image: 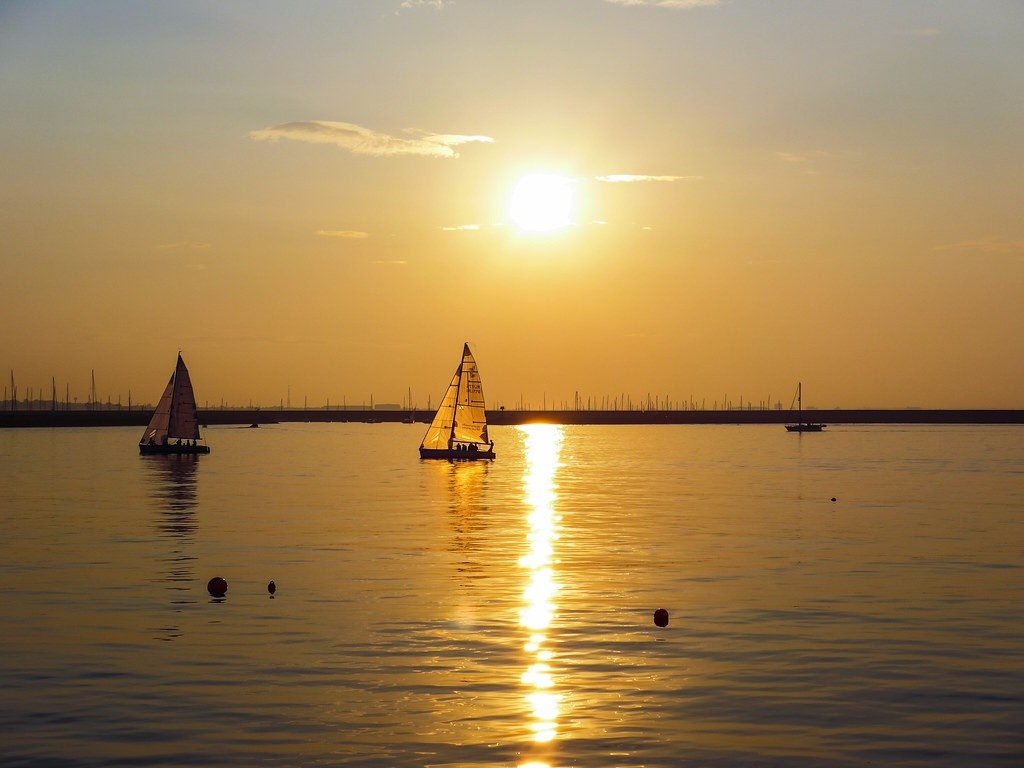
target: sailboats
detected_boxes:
[137,351,212,454]
[418,342,497,461]
[783,381,827,432]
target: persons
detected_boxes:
[488,440,493,452]
[176,438,197,446]
[148,438,158,446]
[456,442,478,452]
